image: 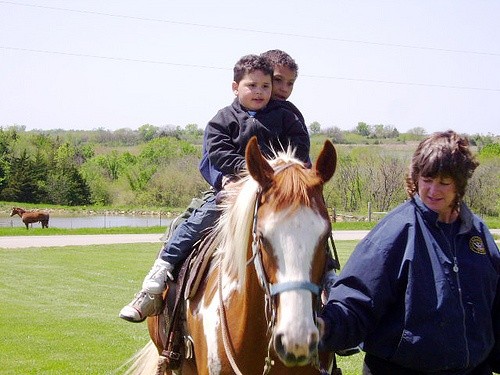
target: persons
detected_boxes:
[160,50,361,356]
[119,55,310,324]
[339,129,500,375]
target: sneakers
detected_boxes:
[141,258,175,294]
[119,293,162,322]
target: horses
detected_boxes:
[10,207,50,229]
[146,136,339,374]
